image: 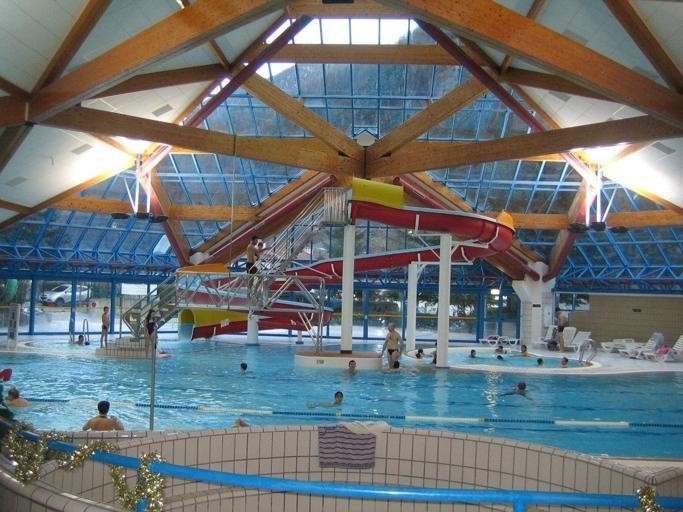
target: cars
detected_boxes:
[40,283,94,306]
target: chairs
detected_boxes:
[479,325,683,362]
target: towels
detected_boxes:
[343,421,390,436]
[318,423,376,469]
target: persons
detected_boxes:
[82,400,123,433]
[380,322,403,368]
[388,361,403,372]
[552,306,568,352]
[520,344,527,357]
[415,351,425,359]
[496,345,505,354]
[142,309,158,358]
[332,392,343,406]
[560,357,567,368]
[470,350,476,358]
[1,387,28,406]
[430,342,436,364]
[257,242,264,258]
[494,383,538,408]
[245,236,272,300]
[238,362,247,373]
[536,359,545,368]
[73,334,84,346]
[99,307,110,348]
[345,360,356,373]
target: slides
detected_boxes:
[177,176,515,341]
